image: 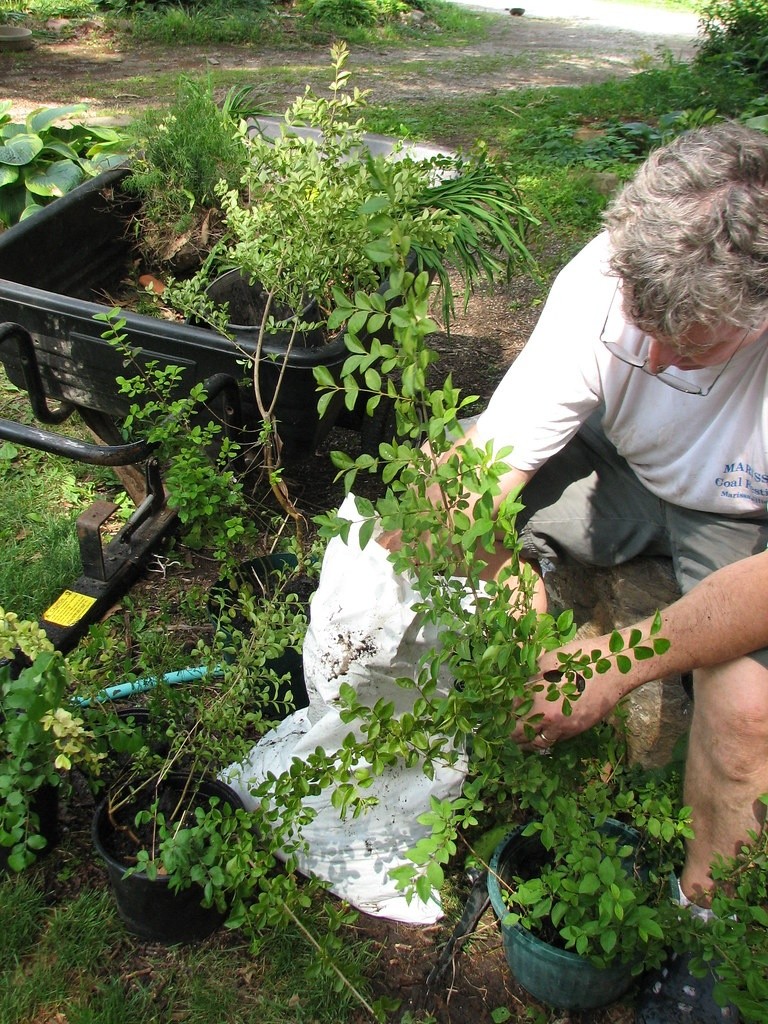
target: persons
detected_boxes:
[375,118,768,1024]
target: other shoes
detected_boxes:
[633,924,740,1023]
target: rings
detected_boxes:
[539,733,556,743]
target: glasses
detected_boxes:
[598,268,757,396]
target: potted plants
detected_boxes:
[0,40,768,1024]
